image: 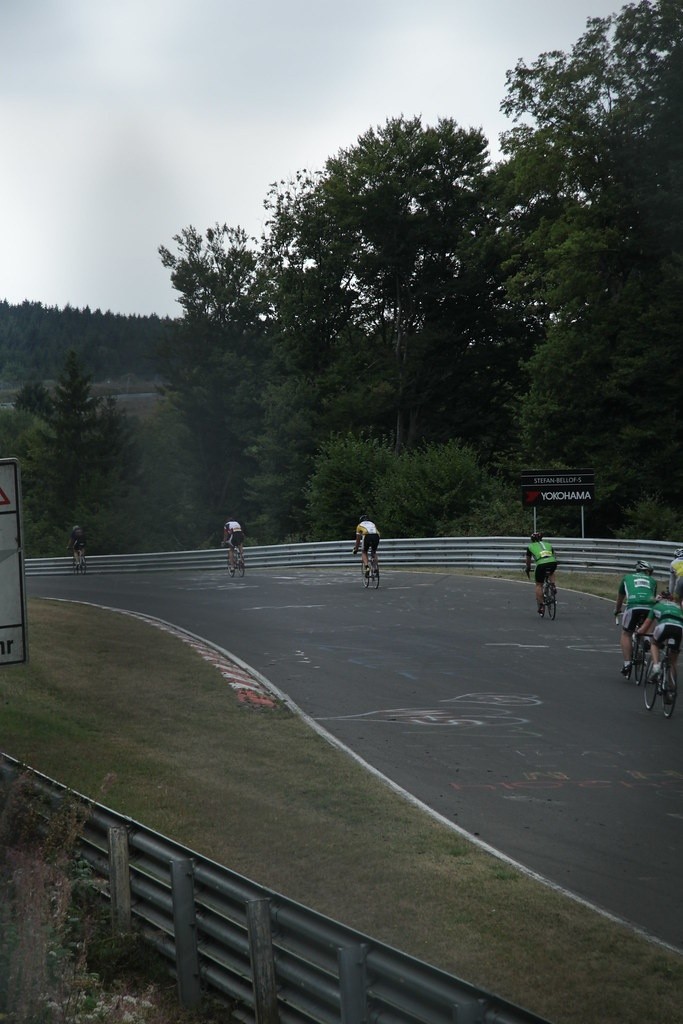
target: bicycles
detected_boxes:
[353,546,380,589]
[525,569,557,620]
[661,586,674,602]
[632,631,680,718]
[221,542,246,578]
[67,547,87,575]
[615,613,651,685]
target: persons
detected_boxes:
[612,558,660,675]
[633,590,683,677]
[525,532,559,615]
[668,548,683,604]
[222,516,245,573]
[354,513,381,580]
[67,525,88,572]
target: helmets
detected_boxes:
[226,518,234,522]
[72,525,80,531]
[674,549,683,559]
[654,591,674,602]
[635,560,654,572]
[359,515,368,522]
[531,533,542,542]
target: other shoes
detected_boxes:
[550,583,557,594]
[76,562,80,569]
[644,640,650,651]
[619,662,633,675]
[664,692,675,705]
[649,665,660,680]
[230,566,235,572]
[364,565,370,577]
[538,603,545,614]
